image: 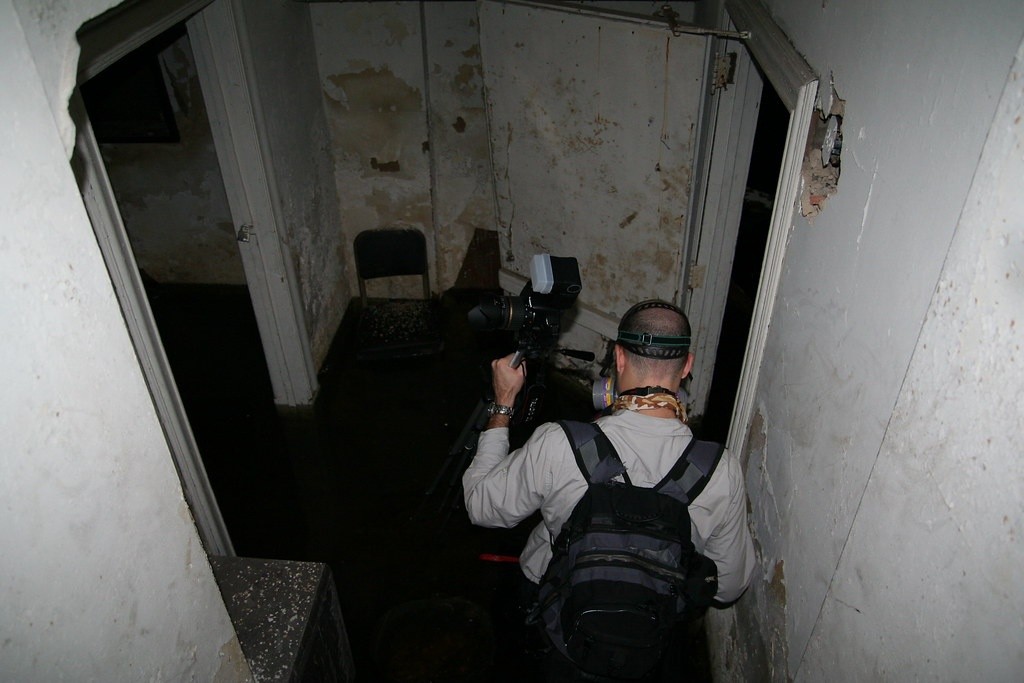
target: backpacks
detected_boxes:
[522,418,725,683]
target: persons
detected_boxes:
[462,300,754,683]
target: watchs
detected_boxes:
[485,402,514,419]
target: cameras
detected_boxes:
[467,253,583,341]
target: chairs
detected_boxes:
[352,224,446,375]
